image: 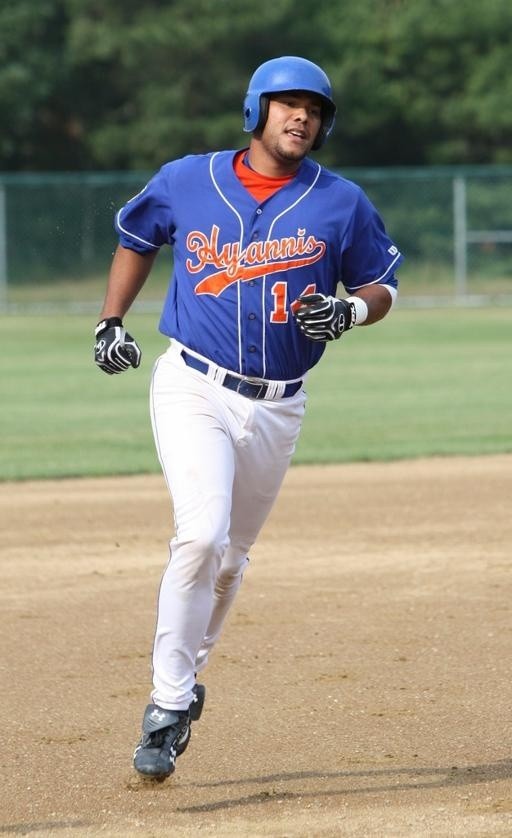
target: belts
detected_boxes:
[181,350,302,400]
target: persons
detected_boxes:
[95,56,404,783]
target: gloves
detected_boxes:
[94,316,142,375]
[295,293,357,342]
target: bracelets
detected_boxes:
[345,296,368,326]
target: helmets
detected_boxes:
[243,56,337,151]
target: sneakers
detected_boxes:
[133,684,206,778]
